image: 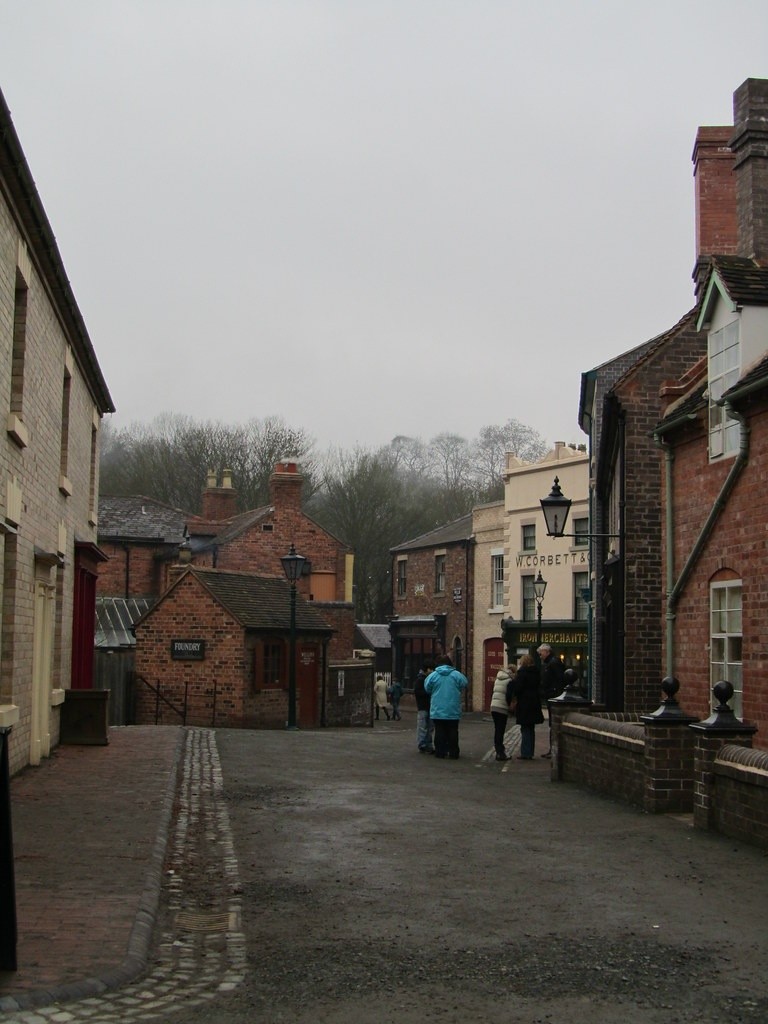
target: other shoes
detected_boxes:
[541,750,551,757]
[449,753,459,759]
[391,717,395,720]
[496,753,511,760]
[387,716,390,721]
[419,747,435,754]
[375,718,379,720]
[398,717,401,721]
[435,754,445,758]
[517,755,533,760]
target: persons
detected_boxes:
[490,655,544,760]
[389,678,404,721]
[374,676,390,720]
[414,656,468,760]
[537,643,566,758]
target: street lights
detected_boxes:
[279,543,306,724]
[532,570,547,678]
